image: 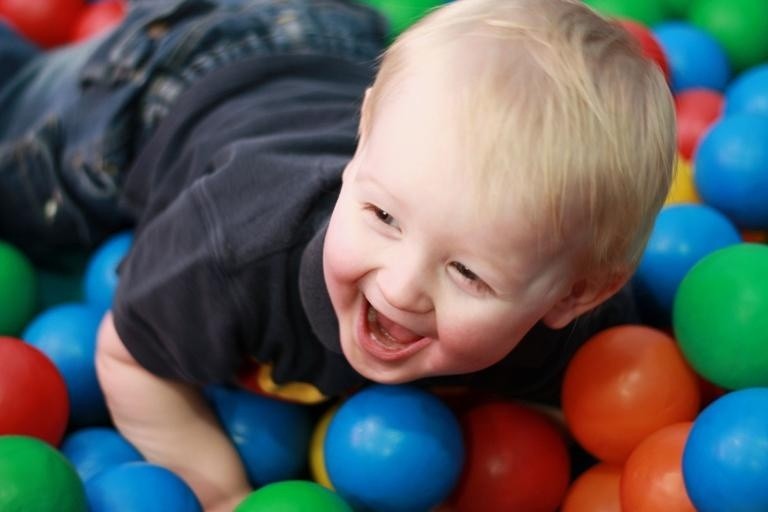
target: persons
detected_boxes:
[85,461,202,512]
[0,0,677,512]
